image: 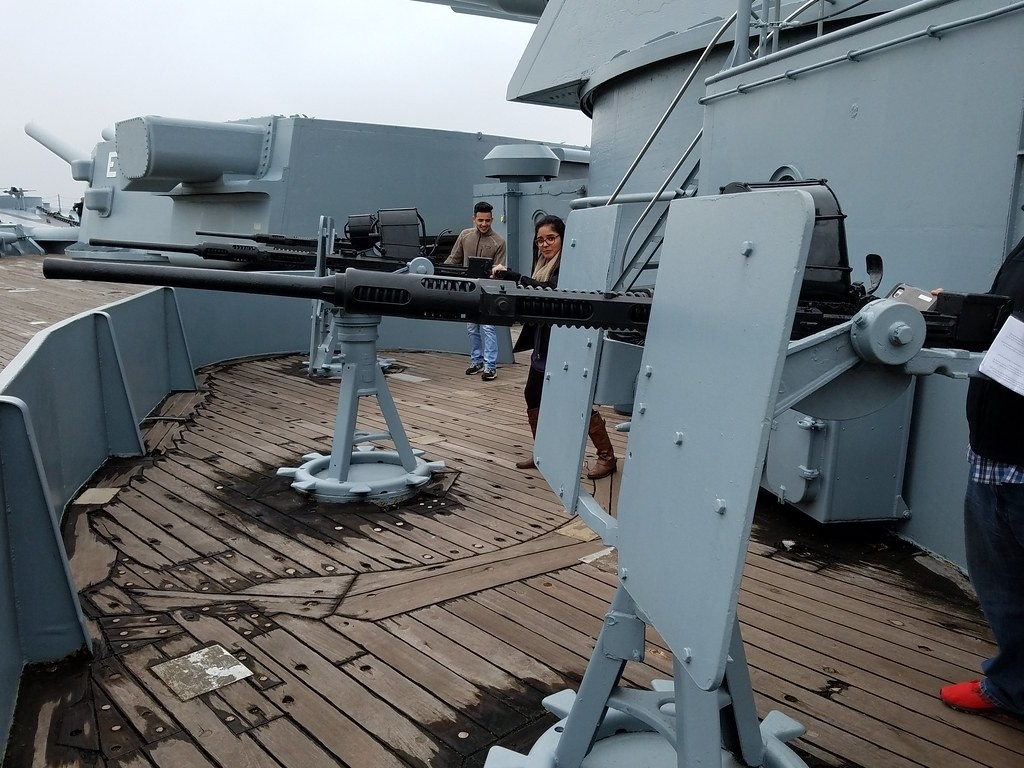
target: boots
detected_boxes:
[587,411,617,479]
[515,408,539,469]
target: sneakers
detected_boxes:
[482,368,498,380]
[466,362,484,375]
[938,679,1023,716]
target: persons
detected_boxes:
[490,215,618,479]
[930,237,1024,715]
[443,202,507,380]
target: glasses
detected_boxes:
[535,234,560,245]
